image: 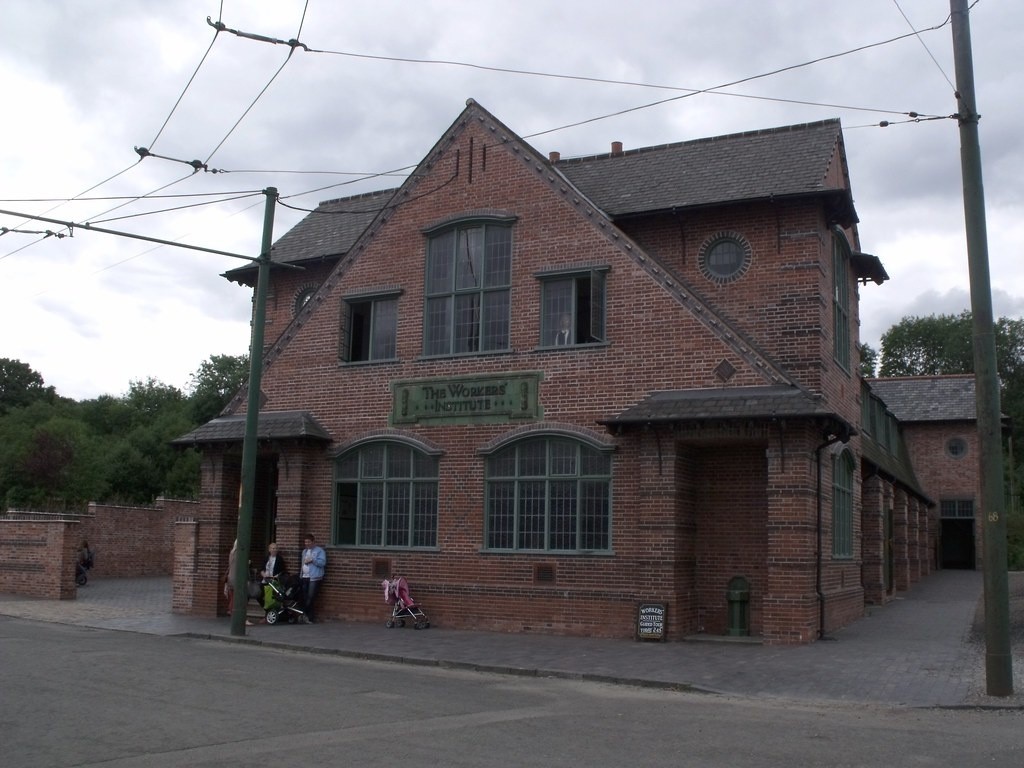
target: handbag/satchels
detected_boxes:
[263,585,275,610]
[248,582,262,598]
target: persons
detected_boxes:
[227,538,255,626]
[297,534,326,625]
[259,541,289,625]
[76,540,93,570]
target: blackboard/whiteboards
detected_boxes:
[639,602,666,640]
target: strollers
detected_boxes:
[382,573,433,630]
[75,541,95,584]
[254,576,310,625]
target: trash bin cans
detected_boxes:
[727,590,751,636]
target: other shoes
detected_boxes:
[245,620,254,625]
[259,618,268,625]
[297,613,310,626]
